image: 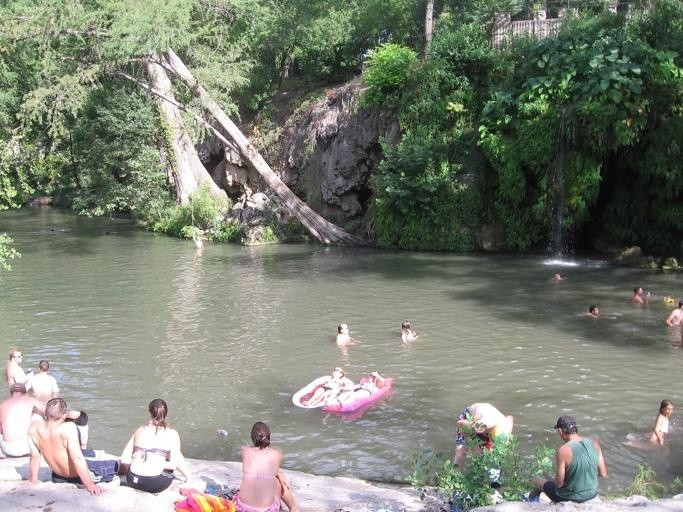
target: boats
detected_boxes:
[290,374,355,410]
[323,376,394,414]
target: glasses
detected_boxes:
[10,355,23,359]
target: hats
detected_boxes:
[553,416,576,430]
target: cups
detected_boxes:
[27,368,32,374]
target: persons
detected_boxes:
[0,348,193,495]
[299,366,385,410]
[527,415,607,504]
[454,403,514,475]
[335,323,355,346]
[400,320,419,343]
[586,305,600,318]
[630,287,650,304]
[665,300,683,326]
[649,399,674,445]
[550,272,563,280]
[232,421,300,512]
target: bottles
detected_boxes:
[216,429,228,438]
